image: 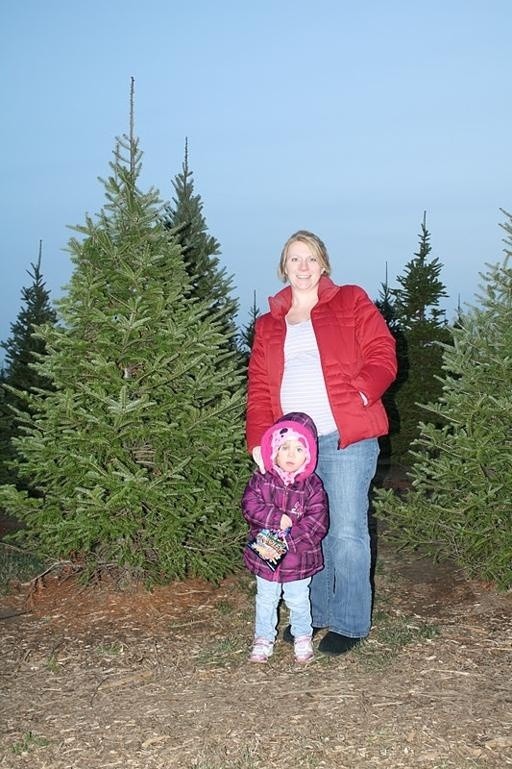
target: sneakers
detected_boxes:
[294,636,314,662]
[318,631,364,656]
[283,623,321,642]
[249,637,276,663]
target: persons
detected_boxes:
[244,229,401,659]
[240,411,330,667]
